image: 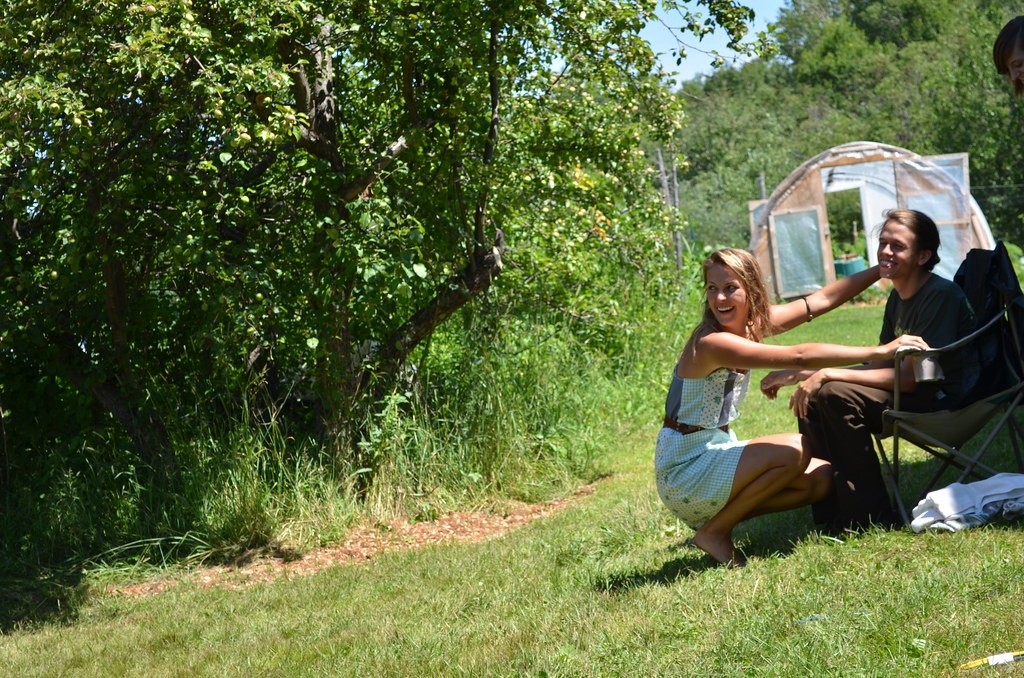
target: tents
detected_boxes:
[751,139,995,303]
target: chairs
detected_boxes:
[871,241,1024,539]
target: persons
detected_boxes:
[761,210,976,535]
[655,248,930,569]
